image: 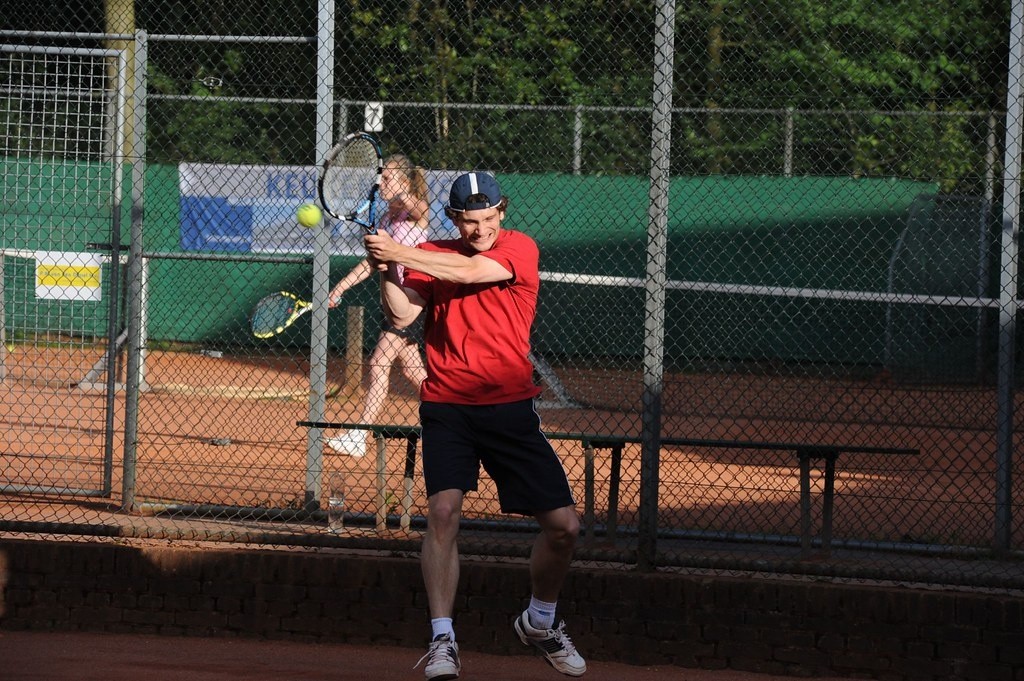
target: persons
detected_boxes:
[321,153,430,458]
[364,172,588,681]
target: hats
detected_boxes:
[448,171,502,211]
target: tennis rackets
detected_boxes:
[252,292,339,338]
[319,131,388,268]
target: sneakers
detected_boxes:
[324,431,366,458]
[424,634,461,680]
[512,609,587,676]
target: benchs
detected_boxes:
[296,419,922,567]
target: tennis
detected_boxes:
[298,203,320,227]
